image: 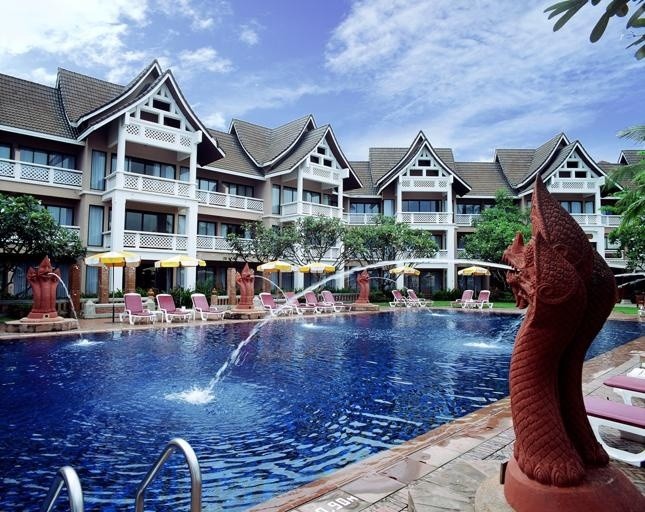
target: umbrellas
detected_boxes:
[154,255,207,308]
[256,260,294,307]
[457,266,492,300]
[84,250,142,322]
[389,266,421,289]
[298,262,335,300]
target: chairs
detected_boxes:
[450,290,493,309]
[259,289,353,317]
[120,291,225,325]
[389,289,433,308]
